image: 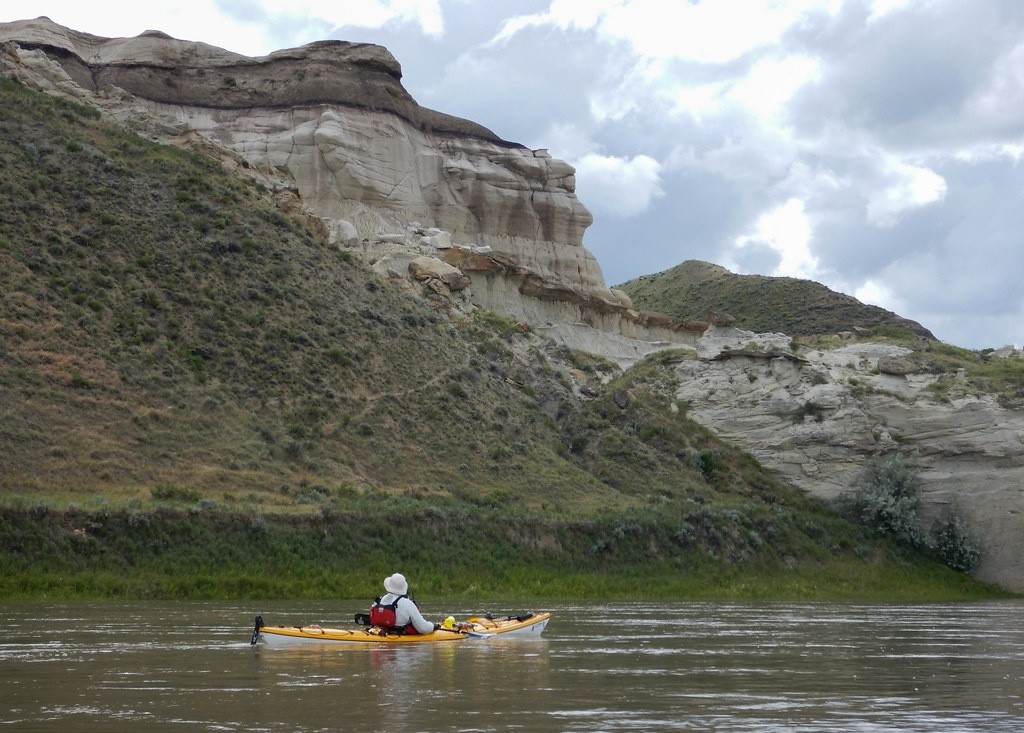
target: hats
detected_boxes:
[384,573,408,596]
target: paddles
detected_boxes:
[353,614,498,639]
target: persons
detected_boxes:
[370,573,441,636]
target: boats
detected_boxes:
[253,613,553,648]
